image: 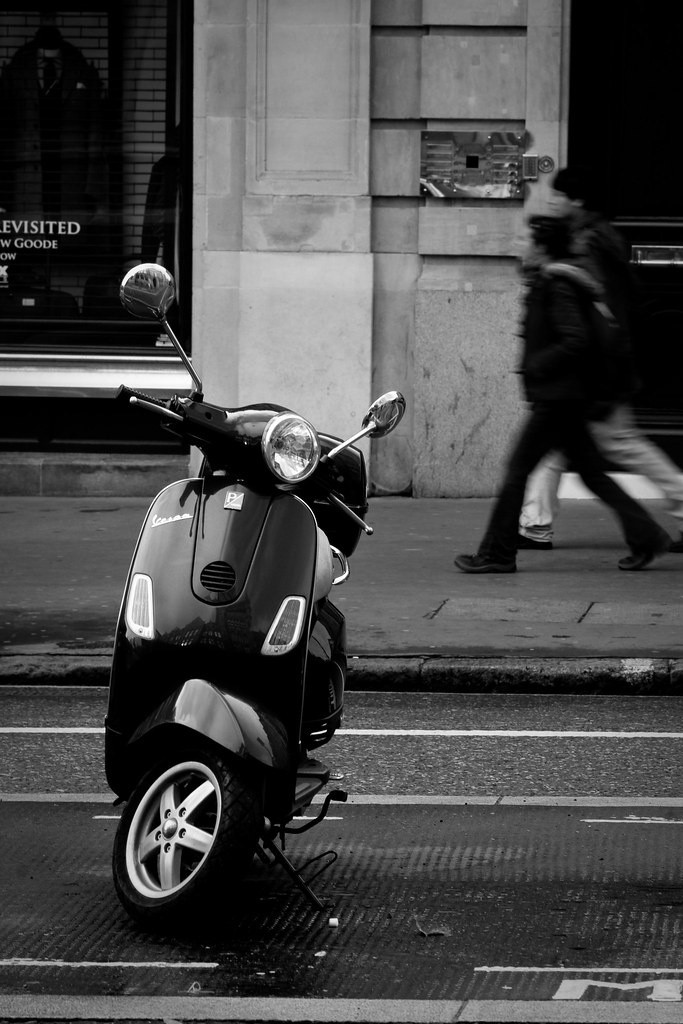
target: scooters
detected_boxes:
[99,261,407,919]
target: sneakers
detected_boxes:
[454,539,521,576]
[617,528,672,573]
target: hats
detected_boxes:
[551,166,606,202]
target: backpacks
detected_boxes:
[533,260,639,425]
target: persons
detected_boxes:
[513,168,683,553]
[452,213,671,573]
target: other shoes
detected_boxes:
[670,523,682,556]
[514,524,557,550]
